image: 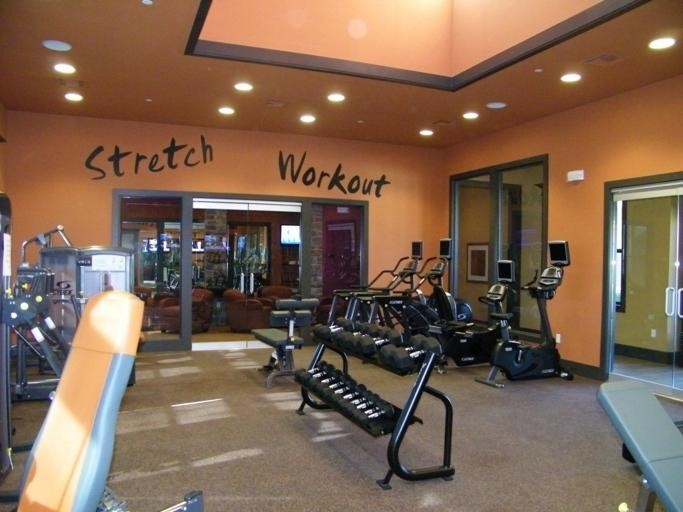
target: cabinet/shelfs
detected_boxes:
[295,319,455,491]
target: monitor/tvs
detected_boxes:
[411,239,423,261]
[496,259,516,283]
[547,241,570,265]
[439,238,452,259]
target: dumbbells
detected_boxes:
[296,360,394,425]
[312,317,442,368]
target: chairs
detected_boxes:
[129,280,352,333]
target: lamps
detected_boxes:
[186,196,306,215]
